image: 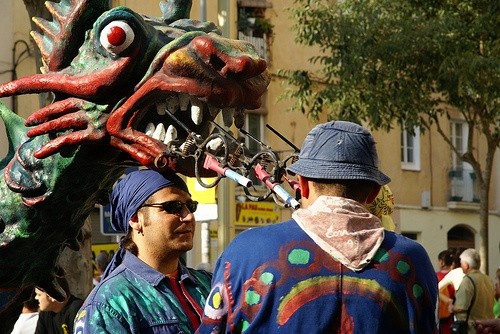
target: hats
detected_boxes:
[285,121,392,185]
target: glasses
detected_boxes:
[140,200,198,214]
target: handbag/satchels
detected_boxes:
[451,320,467,334]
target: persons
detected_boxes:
[75,168,213,334]
[194,121,438,334]
[435,248,500,334]
[10,216,115,334]
